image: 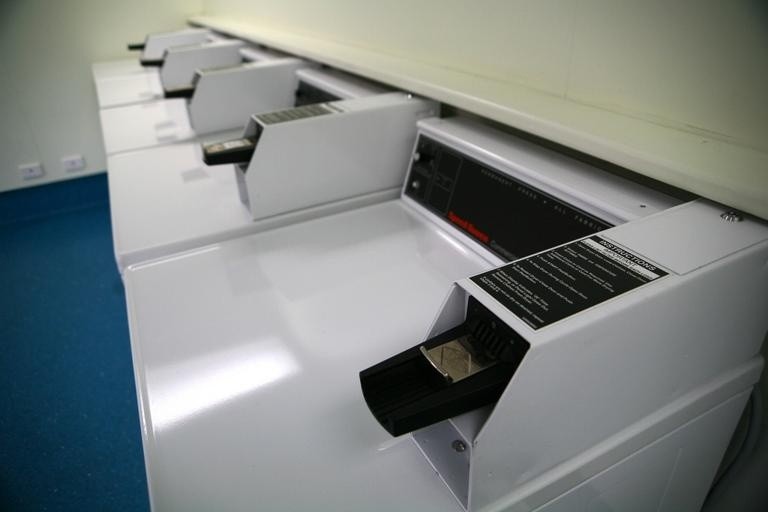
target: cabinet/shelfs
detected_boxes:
[106,67,442,274]
[92,27,218,79]
[99,47,311,159]
[94,35,245,110]
[122,114,768,512]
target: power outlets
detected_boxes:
[19,162,44,180]
[61,155,84,173]
